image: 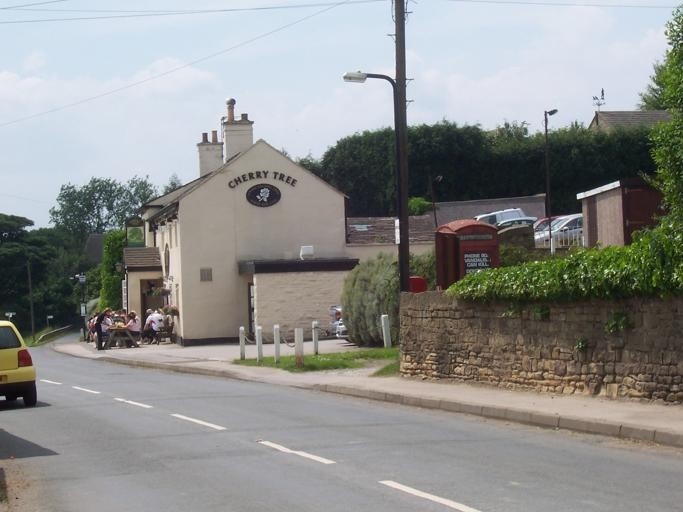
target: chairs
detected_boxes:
[157,322,175,344]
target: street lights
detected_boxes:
[544,108,557,214]
[342,70,410,291]
[78,273,86,341]
[115,262,128,315]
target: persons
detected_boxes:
[84,306,172,350]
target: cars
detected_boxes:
[330,304,350,339]
[476,208,584,248]
[1,319,37,406]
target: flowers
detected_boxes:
[256,187,269,202]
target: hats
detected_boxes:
[146,308,153,313]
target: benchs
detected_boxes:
[99,334,140,350]
